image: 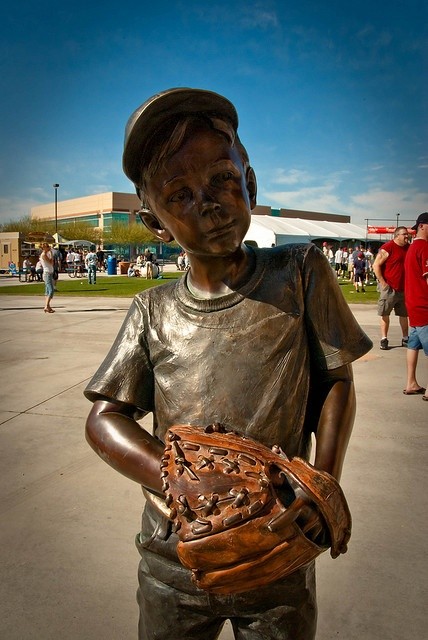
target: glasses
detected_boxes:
[45,245,49,248]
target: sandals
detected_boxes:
[43,308,54,313]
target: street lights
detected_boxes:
[396,213,400,228]
[53,183,59,233]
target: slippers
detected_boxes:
[422,395,428,400]
[402,386,426,394]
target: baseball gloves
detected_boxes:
[161,425,353,598]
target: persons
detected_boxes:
[46,249,53,260]
[126,248,160,280]
[85,86,371,637]
[39,242,55,313]
[322,240,374,291]
[176,251,188,272]
[23,255,33,281]
[403,212,428,402]
[372,226,410,351]
[35,258,44,282]
[66,248,83,277]
[49,243,62,292]
[84,245,98,284]
[96,247,105,273]
[28,265,38,282]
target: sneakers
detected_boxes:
[362,288,365,292]
[356,288,359,292]
[401,336,409,346]
[379,338,389,350]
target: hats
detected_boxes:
[323,242,327,246]
[411,212,428,230]
[358,252,363,258]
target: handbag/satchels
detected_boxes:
[53,255,58,281]
